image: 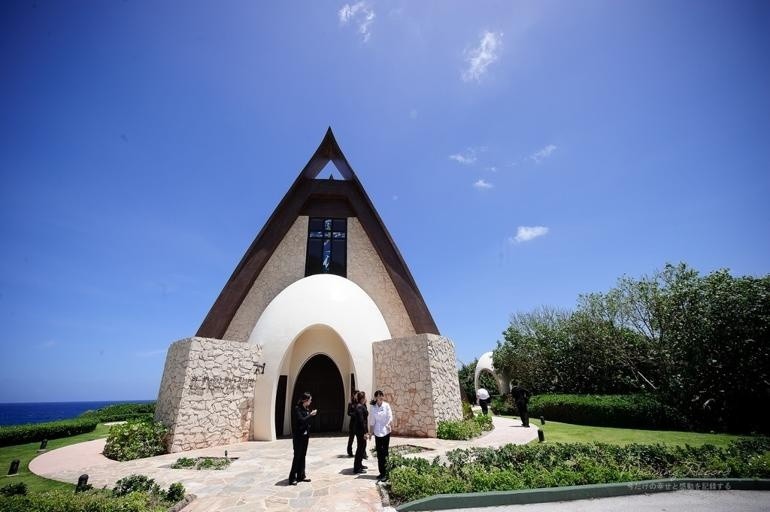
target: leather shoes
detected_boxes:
[360,466,368,469]
[289,477,298,485]
[348,453,355,457]
[298,477,312,482]
[376,473,388,482]
[362,454,368,459]
[354,469,367,474]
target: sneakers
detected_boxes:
[521,424,530,427]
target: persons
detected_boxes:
[368,390,393,482]
[289,391,318,485]
[512,379,530,427]
[353,392,368,474]
[347,389,369,460]
[477,384,491,415]
